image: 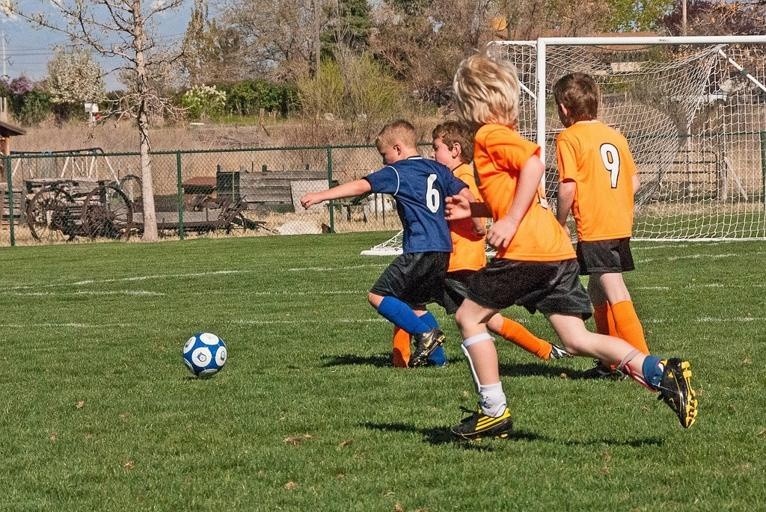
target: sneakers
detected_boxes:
[582,363,618,379]
[392,328,446,367]
[657,356,699,428]
[449,400,516,443]
[550,343,573,360]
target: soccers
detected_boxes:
[183,332,227,378]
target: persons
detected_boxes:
[391,119,572,370]
[443,54,698,436]
[551,72,653,387]
[299,118,485,370]
